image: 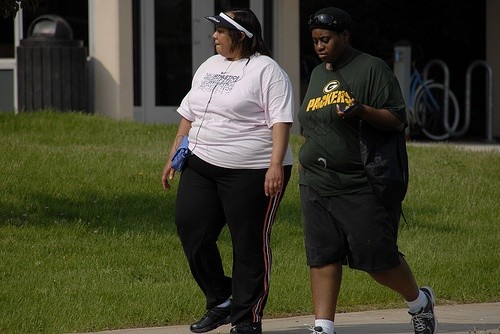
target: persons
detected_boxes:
[297,6,440,334]
[161,6,296,334]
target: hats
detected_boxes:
[204,12,253,38]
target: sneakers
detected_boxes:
[308,327,336,334]
[230,323,262,334]
[408,286,437,334]
[190,299,233,333]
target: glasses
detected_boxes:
[308,13,346,29]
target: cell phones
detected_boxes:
[341,103,355,113]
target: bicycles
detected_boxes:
[408,58,459,141]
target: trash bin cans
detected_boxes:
[16,15,84,115]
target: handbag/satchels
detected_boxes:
[171,136,189,172]
[360,119,408,204]
